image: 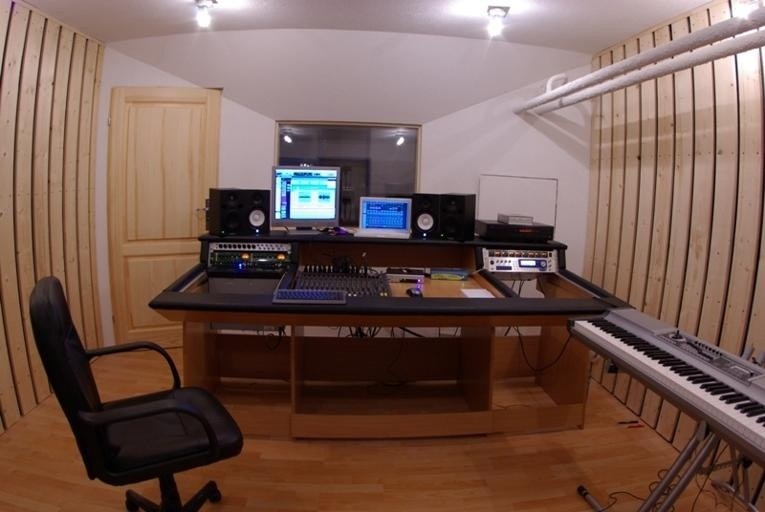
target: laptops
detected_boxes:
[354,195,413,239]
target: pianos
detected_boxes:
[567,306,765,468]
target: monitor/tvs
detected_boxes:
[269,165,341,235]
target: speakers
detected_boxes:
[208,187,269,236]
[412,192,476,242]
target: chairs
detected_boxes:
[28,277,242,511]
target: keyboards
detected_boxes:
[272,288,347,304]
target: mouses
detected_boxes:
[406,287,423,298]
